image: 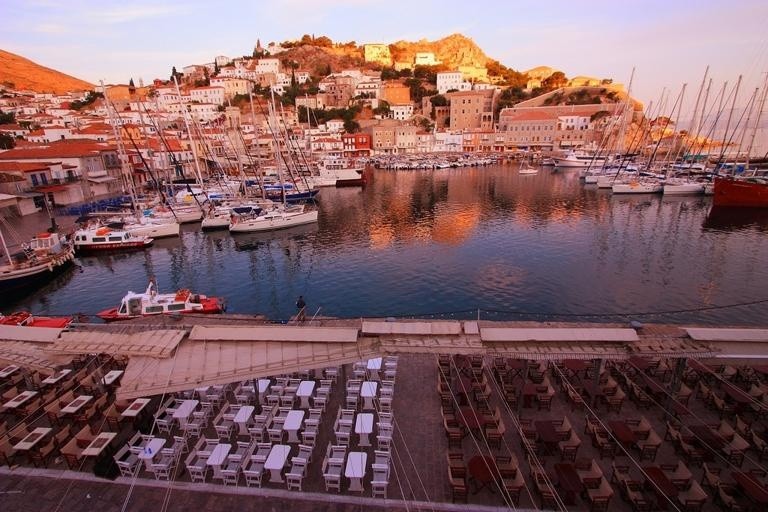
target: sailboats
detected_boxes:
[0,201,75,282]
[70,75,370,251]
[539,72,768,208]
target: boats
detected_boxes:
[91,281,224,318]
[518,153,538,175]
[0,310,75,329]
[374,153,499,170]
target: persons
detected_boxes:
[295,295,307,320]
[147,274,157,297]
[207,203,215,216]
